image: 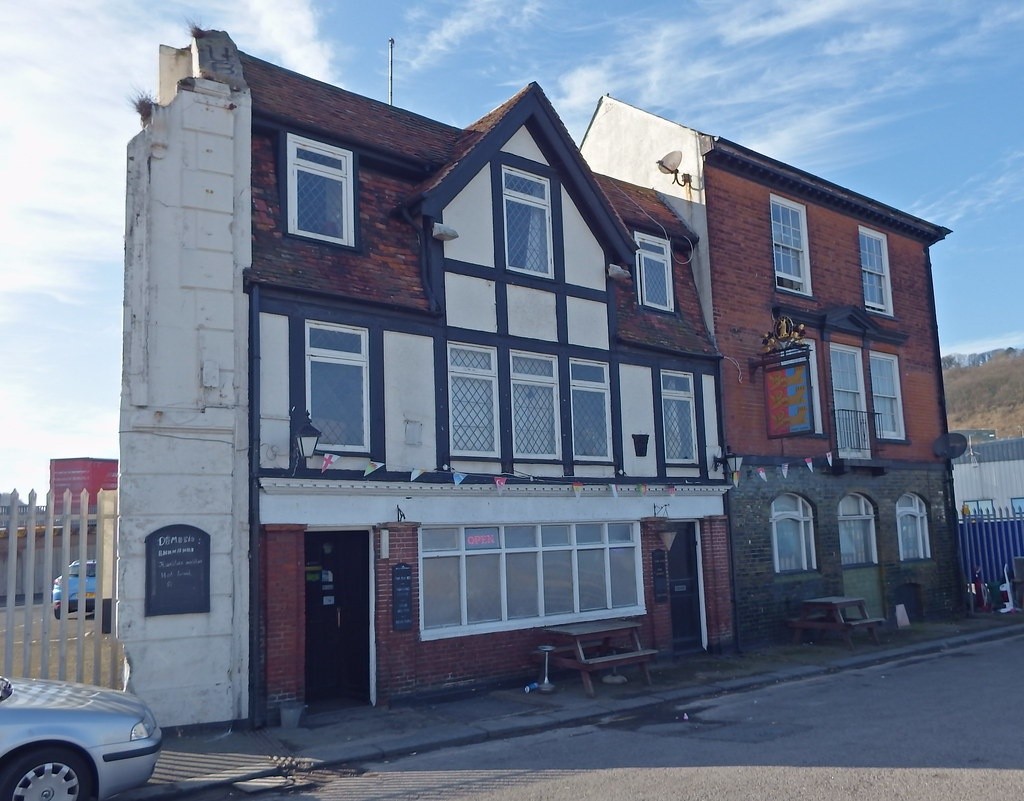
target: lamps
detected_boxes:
[380,528,390,560]
[605,264,632,282]
[713,445,743,484]
[657,149,689,186]
[431,220,460,242]
[294,410,321,459]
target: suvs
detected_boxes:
[51,559,96,620]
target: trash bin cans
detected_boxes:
[1012,557,1024,613]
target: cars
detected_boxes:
[0,674,165,801]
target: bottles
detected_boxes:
[525,682,539,693]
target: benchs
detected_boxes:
[530,643,659,672]
[780,614,887,633]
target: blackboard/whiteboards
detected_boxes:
[143,523,212,617]
[391,562,412,631]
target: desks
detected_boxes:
[539,622,657,696]
[790,597,880,651]
